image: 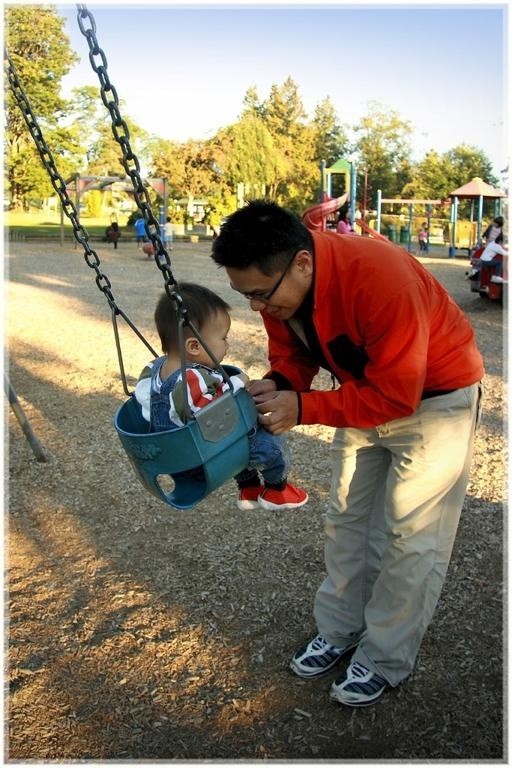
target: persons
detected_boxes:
[134,216,147,250]
[208,198,486,712]
[132,280,310,511]
[480,233,508,286]
[336,205,358,235]
[416,227,428,257]
[481,216,504,244]
[420,222,430,257]
[110,212,121,249]
[145,219,157,259]
[162,216,174,250]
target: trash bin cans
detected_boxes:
[388,224,408,242]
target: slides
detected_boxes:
[300,192,349,233]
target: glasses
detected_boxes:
[229,269,290,302]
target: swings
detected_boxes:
[5,5,262,512]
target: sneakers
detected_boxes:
[257,482,309,510]
[329,658,390,708]
[237,484,264,512]
[289,629,368,680]
